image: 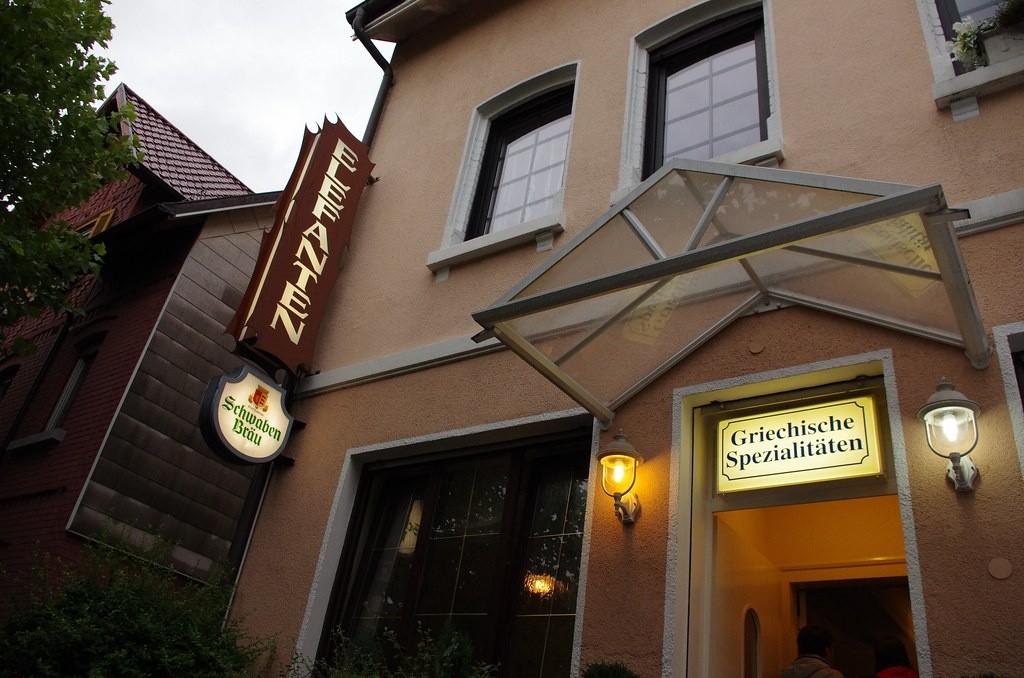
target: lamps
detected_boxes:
[916,374,982,493]
[595,424,642,526]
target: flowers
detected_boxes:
[953,0,1024,72]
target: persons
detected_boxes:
[778,625,844,678]
[873,635,919,678]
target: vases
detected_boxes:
[978,23,1024,65]
